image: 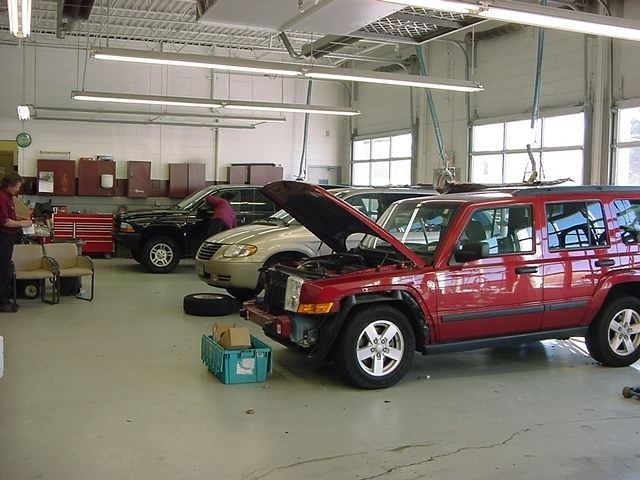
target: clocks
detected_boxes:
[16,133,33,148]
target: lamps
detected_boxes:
[17,41,31,121]
[390,0,639,43]
[88,1,485,93]
[8,1,32,39]
[70,26,361,117]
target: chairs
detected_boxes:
[10,245,56,311]
[462,221,489,257]
[43,243,94,304]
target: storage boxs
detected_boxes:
[220,329,252,350]
[200,332,272,384]
[211,322,236,342]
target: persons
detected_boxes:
[0,171,32,312]
[206,190,238,237]
